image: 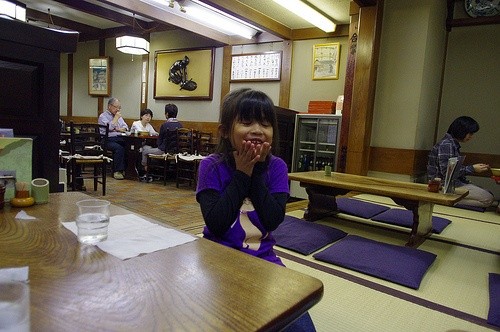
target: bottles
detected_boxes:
[428,177,442,192]
[0,183,6,213]
[130,125,135,137]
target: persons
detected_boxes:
[98,98,128,179]
[140,104,182,182]
[426,115,494,206]
[130,109,157,173]
[197,87,288,267]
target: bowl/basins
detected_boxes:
[490,168,500,176]
[137,129,150,136]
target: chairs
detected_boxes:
[60,119,218,196]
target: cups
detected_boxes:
[0,279,31,332]
[75,200,111,245]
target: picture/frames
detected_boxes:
[230,50,282,82]
[153,46,216,100]
[312,42,341,80]
[87,56,111,96]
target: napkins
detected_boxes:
[63,212,197,261]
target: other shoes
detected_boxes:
[129,174,141,181]
[113,172,124,179]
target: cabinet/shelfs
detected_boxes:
[291,113,342,171]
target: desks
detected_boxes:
[117,134,159,182]
[288,170,469,246]
[0,190,324,332]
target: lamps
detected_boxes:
[155,0,337,39]
[0,0,26,22]
[115,14,149,56]
